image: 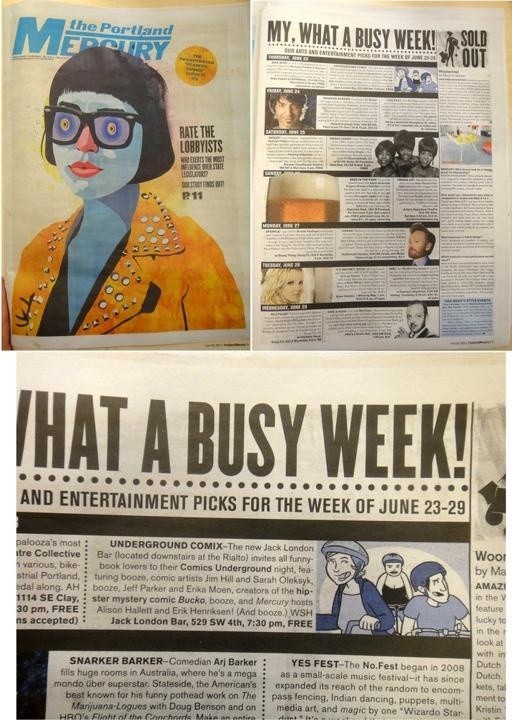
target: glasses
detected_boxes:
[44,107,140,149]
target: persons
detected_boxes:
[408,223,435,267]
[394,300,438,337]
[390,130,419,177]
[394,66,437,94]
[404,136,440,178]
[263,266,306,308]
[443,29,458,66]
[370,141,399,176]
[11,47,252,338]
[271,92,309,133]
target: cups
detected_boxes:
[266,174,341,223]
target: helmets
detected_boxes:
[321,540,370,566]
[410,561,447,593]
[382,553,405,566]
